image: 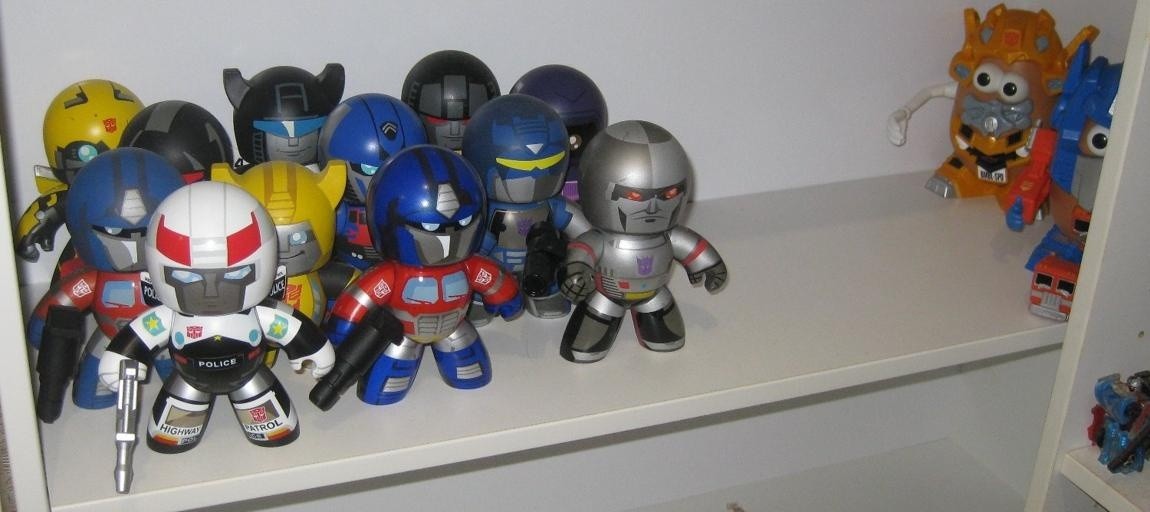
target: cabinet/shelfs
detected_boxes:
[1027,0,1149,512]
[0,0,1142,512]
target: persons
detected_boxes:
[3,47,730,495]
